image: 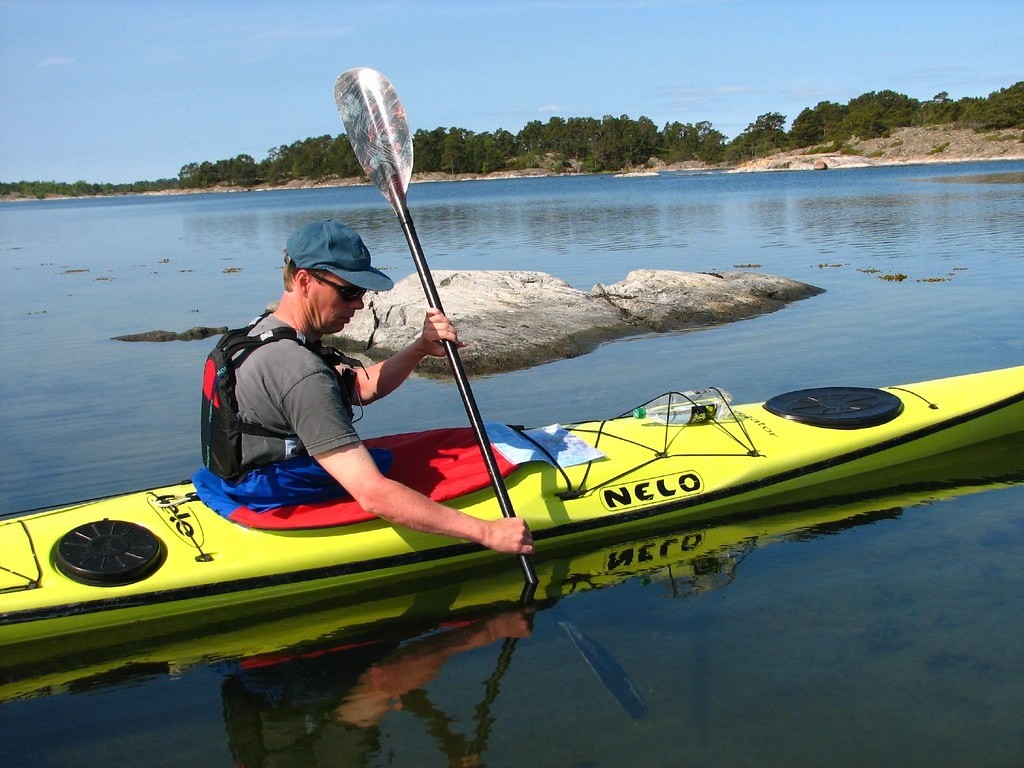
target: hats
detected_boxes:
[286,219,394,293]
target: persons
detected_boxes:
[237,607,534,768]
[201,219,537,554]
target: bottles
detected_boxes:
[634,386,734,427]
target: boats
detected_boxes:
[0,432,1024,706]
[0,363,1023,702]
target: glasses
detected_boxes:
[306,271,367,303]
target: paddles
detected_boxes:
[332,67,538,589]
